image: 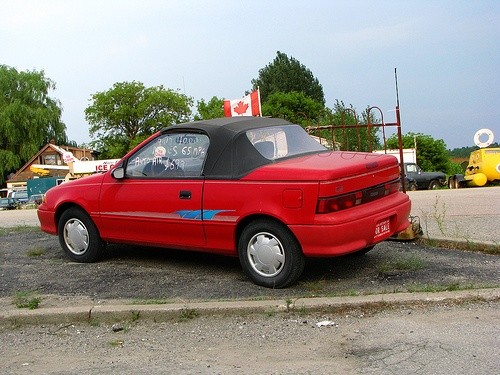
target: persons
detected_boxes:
[141,145,169,177]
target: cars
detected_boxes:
[35,116,412,289]
[397,162,447,191]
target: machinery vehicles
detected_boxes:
[448,147,500,189]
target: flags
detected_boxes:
[223,88,261,118]
